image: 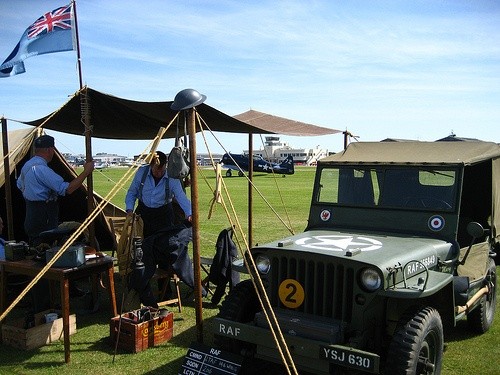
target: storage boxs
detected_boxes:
[5,241,27,263]
[109,309,173,353]
[46,246,88,269]
[2,308,76,353]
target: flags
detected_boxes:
[0,2,77,78]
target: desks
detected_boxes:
[0,254,118,362]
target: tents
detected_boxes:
[0,87,352,349]
[0,128,145,276]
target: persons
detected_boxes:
[16,135,95,311]
[125,151,205,302]
[0,218,7,262]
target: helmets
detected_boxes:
[170,89,206,110]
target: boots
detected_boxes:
[138,283,159,309]
[157,278,178,306]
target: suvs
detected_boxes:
[211,134,500,375]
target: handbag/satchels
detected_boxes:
[167,147,190,179]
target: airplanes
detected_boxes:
[219,151,295,177]
[94,162,109,172]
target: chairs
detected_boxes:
[108,215,237,313]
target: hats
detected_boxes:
[35,135,54,148]
